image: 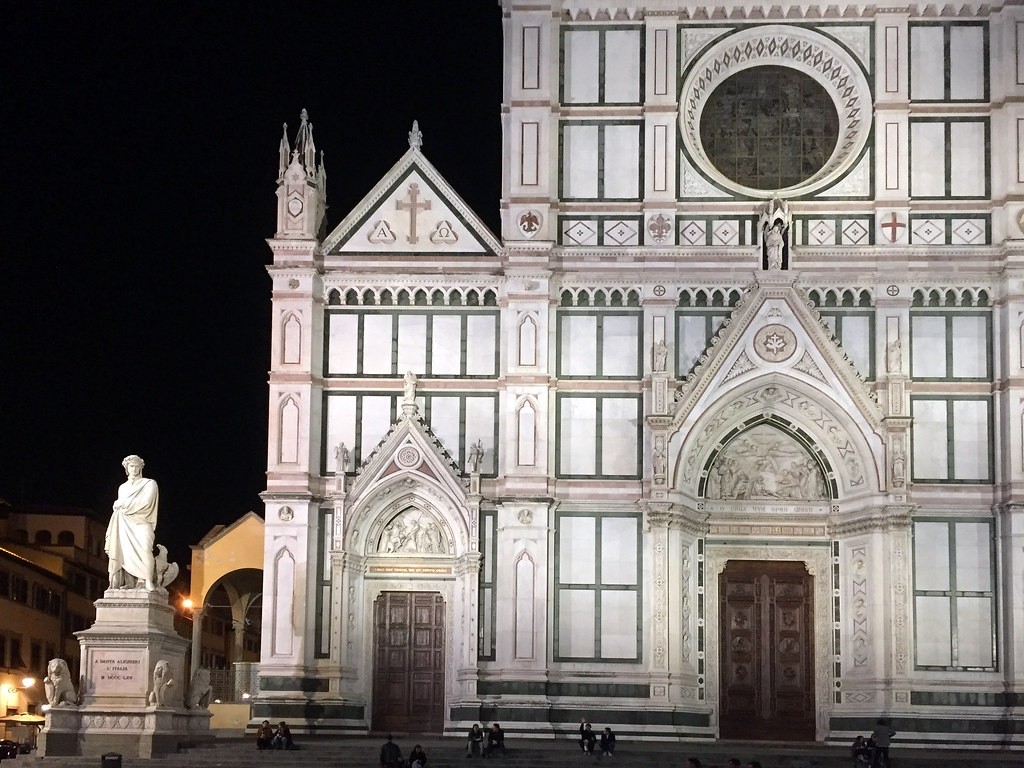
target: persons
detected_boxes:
[334,442,349,471]
[272,721,293,750]
[379,735,404,768]
[409,745,428,768]
[487,723,506,757]
[256,720,273,750]
[850,719,896,768]
[766,226,785,270]
[104,455,159,592]
[466,724,485,759]
[598,727,616,757]
[466,443,483,472]
[578,717,597,756]
[403,371,417,402]
